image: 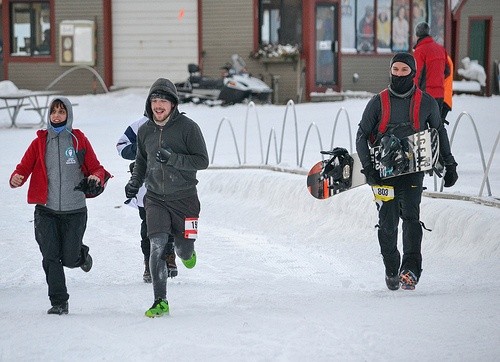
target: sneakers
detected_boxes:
[398,269,417,290]
[385,272,399,290]
[181,250,196,269]
[145,297,169,318]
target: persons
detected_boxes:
[377,6,391,47]
[127,78,209,317]
[359,5,373,45]
[393,5,409,50]
[411,21,454,120]
[116,114,178,283]
[356,53,458,290]
[9,97,111,314]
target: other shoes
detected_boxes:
[167,263,178,277]
[47,302,68,315]
[142,268,152,284]
[80,255,92,272]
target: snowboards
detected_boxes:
[307,128,440,199]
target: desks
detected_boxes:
[0,91,63,129]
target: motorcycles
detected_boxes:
[173,63,272,107]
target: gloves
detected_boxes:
[156,141,175,165]
[73,178,96,196]
[360,164,383,187]
[443,163,458,187]
[125,184,138,198]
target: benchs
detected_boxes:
[0,103,78,110]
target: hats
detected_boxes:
[149,90,175,103]
[390,52,417,72]
[415,22,430,36]
